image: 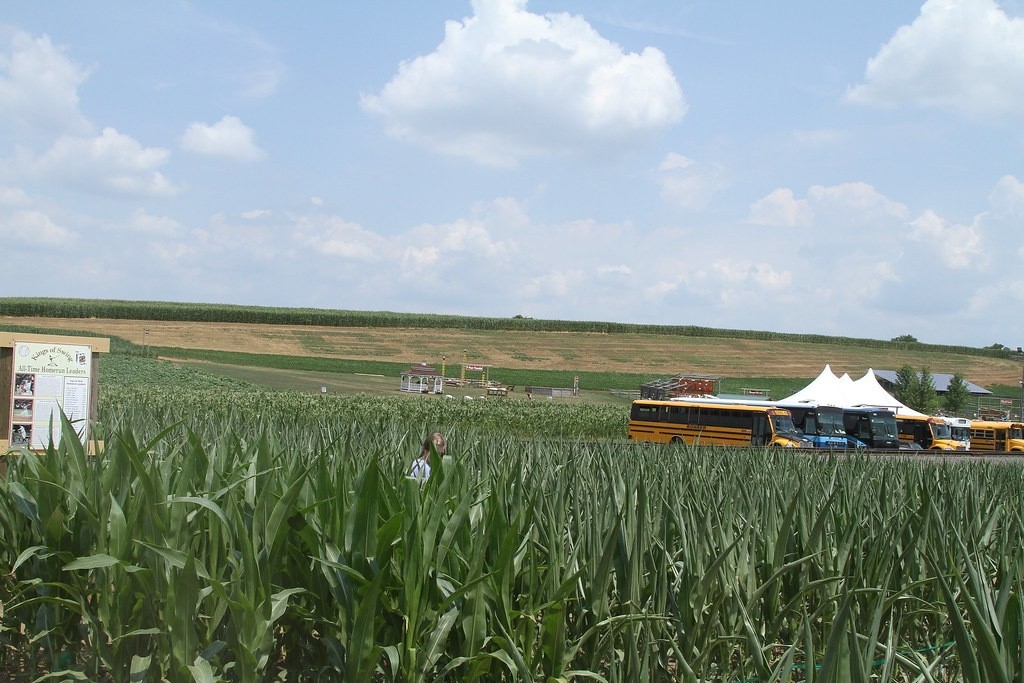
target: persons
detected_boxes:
[1000,411,1007,420]
[936,410,943,417]
[407,432,445,478]
[21,378,27,393]
[21,402,31,417]
[18,425,28,443]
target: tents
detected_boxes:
[782,362,929,416]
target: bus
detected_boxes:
[942,416,974,450]
[627,399,814,449]
[895,415,968,451]
[779,405,847,449]
[843,407,899,449]
[964,420,1024,450]
